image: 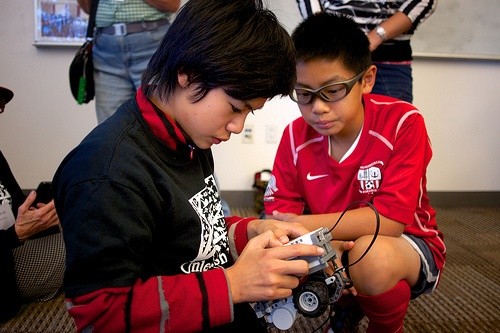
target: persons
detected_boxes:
[264,13,448,333]
[0,148,67,328]
[52,0,325,333]
[42,12,86,37]
[77,0,180,121]
[298,0,437,103]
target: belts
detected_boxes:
[96,19,170,36]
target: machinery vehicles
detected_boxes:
[248,227,346,331]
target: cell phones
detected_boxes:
[29,181,54,210]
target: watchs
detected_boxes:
[375,26,386,41]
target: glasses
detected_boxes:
[289,65,370,105]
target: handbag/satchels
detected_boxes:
[69,40,95,105]
[253,169,273,214]
